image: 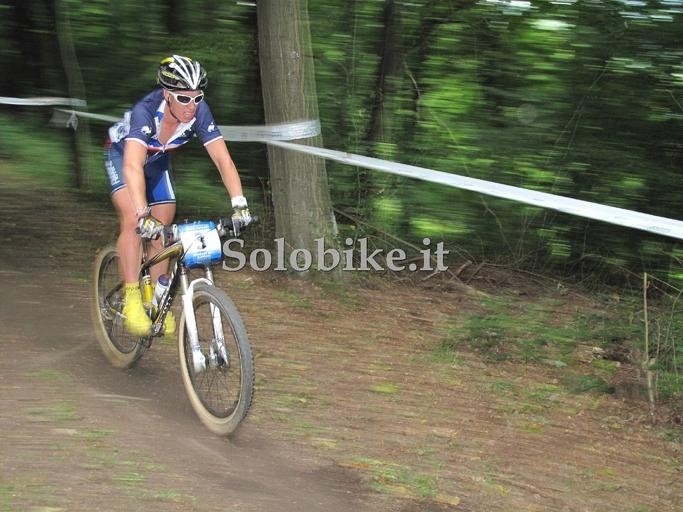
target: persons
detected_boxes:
[103,54,252,337]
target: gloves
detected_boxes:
[231,206,252,237]
[138,214,162,239]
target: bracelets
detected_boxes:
[234,196,247,209]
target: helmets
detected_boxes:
[156,54,208,90]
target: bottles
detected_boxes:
[150,268,169,309]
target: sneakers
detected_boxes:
[146,311,176,335]
[124,304,153,335]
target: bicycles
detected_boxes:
[90,216,261,438]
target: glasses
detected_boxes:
[167,90,204,106]
